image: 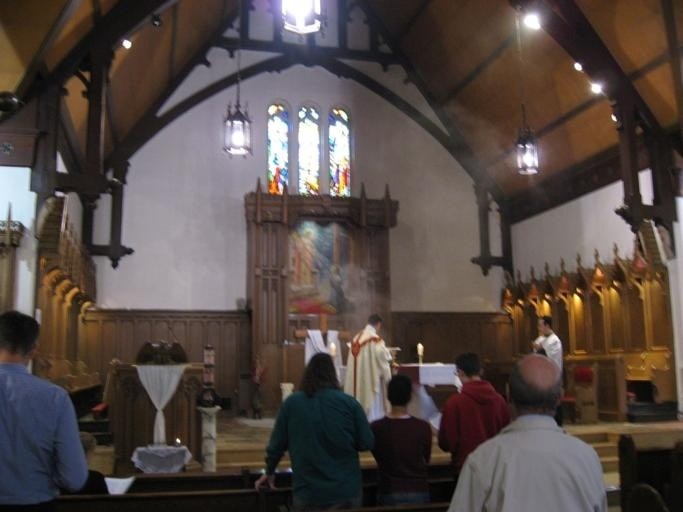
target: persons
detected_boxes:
[437,350,506,479]
[529,316,566,429]
[0,309,88,511]
[346,313,399,422]
[362,372,430,505]
[446,350,609,512]
[251,353,373,512]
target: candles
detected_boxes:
[330,342,336,357]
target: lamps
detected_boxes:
[222,49,254,159]
[515,104,540,177]
